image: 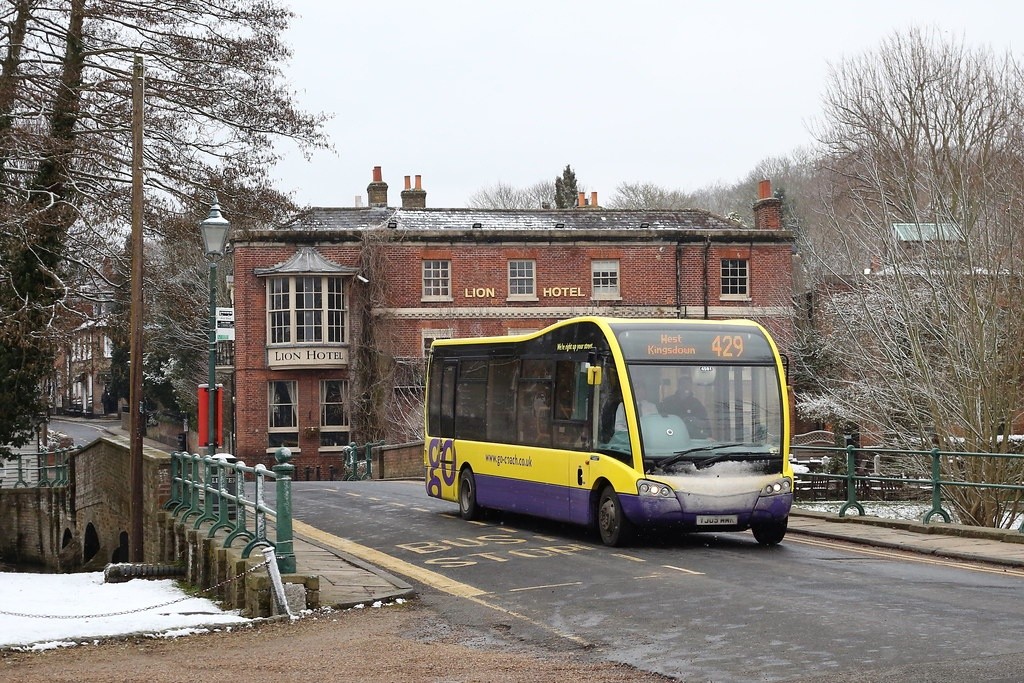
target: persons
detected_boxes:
[615,382,659,434]
[659,375,713,441]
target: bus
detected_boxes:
[413,317,796,550]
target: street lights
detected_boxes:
[195,193,235,456]
